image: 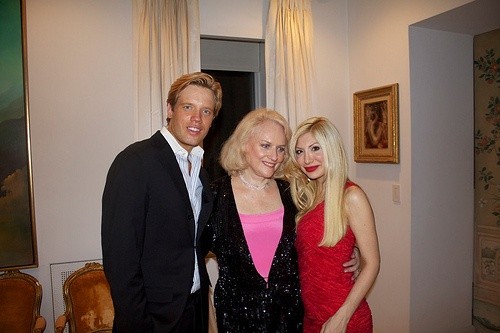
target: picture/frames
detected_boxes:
[354,83,400,164]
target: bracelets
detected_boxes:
[354,245,360,253]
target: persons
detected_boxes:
[283,117,381,333]
[210,108,361,333]
[101,73,222,333]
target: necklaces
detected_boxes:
[237,171,266,191]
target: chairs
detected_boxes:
[55,262,114,333]
[0,268,47,333]
